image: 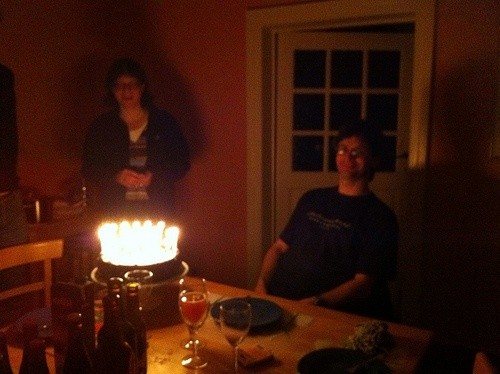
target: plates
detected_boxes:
[211,295,283,327]
[295,345,396,374]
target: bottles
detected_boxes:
[96,297,135,374]
[29,339,51,374]
[104,276,126,374]
[18,321,39,374]
[61,313,95,374]
[0,329,13,374]
[124,282,148,374]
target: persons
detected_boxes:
[0,64,30,292]
[255,119,401,323]
[81,57,190,223]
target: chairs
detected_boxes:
[0,238,66,374]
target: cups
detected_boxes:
[24,198,44,224]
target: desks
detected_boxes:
[40,213,96,276]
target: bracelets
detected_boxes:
[312,294,322,305]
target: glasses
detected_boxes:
[113,81,143,89]
[336,146,370,158]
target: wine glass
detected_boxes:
[178,291,210,368]
[220,300,251,374]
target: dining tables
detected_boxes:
[51,276,433,374]
